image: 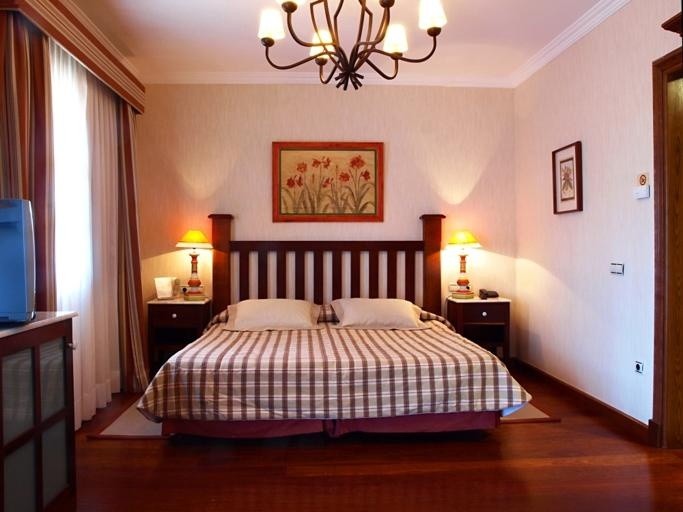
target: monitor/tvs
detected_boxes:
[0,198,36,322]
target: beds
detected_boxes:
[158,211,507,440]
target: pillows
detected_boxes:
[218,296,426,331]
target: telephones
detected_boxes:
[479,289,498,299]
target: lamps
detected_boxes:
[251,1,448,92]
[442,229,482,301]
[174,229,214,301]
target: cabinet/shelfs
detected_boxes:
[0,318,76,512]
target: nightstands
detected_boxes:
[145,295,213,385]
[445,295,511,372]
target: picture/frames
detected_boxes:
[551,141,582,215]
[270,141,384,224]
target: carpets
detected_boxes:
[83,394,561,441]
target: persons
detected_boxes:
[172,279,181,297]
